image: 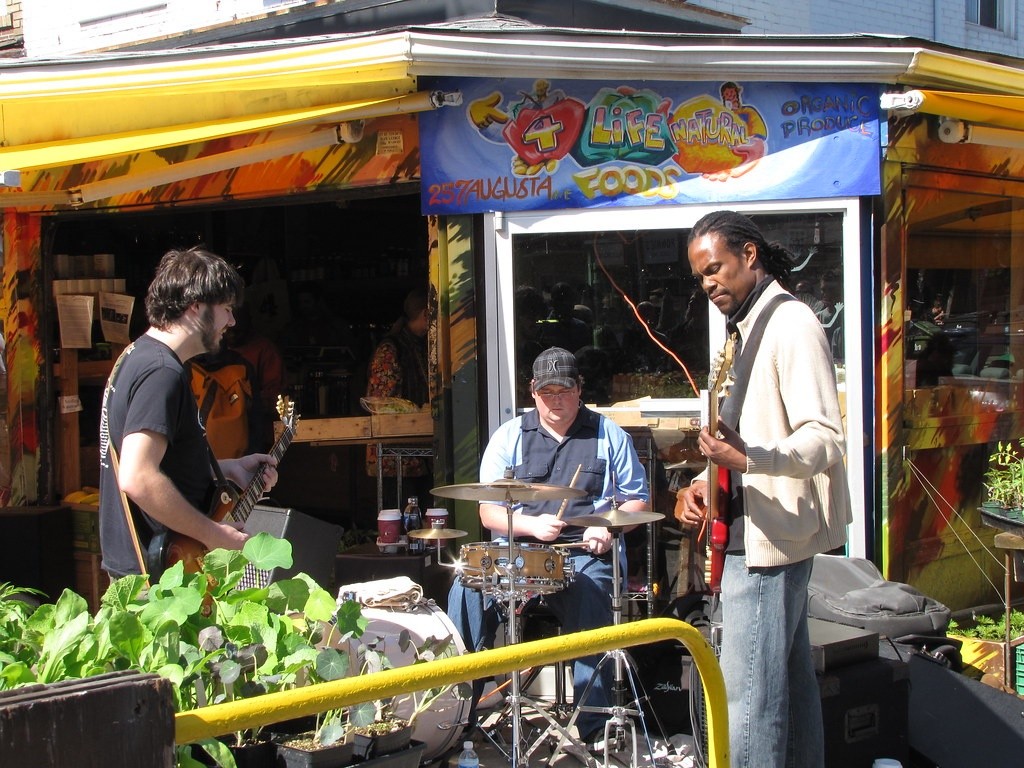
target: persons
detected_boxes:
[363,286,433,534]
[515,267,842,406]
[182,281,356,506]
[673,210,852,768]
[447,345,649,752]
[101,245,287,768]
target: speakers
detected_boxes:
[233,506,344,595]
[698,617,881,768]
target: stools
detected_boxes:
[486,611,575,750]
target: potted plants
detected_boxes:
[981,436,1024,523]
[947,608,1024,691]
[0,532,476,768]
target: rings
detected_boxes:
[601,547,607,552]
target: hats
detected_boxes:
[532,345,581,391]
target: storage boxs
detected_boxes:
[60,501,99,552]
[1015,644,1024,696]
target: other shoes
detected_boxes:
[584,729,626,756]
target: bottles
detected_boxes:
[408,498,423,555]
[404,498,422,534]
[611,370,707,402]
[458,741,479,768]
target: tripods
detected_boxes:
[439,501,604,768]
[545,527,677,768]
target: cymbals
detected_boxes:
[663,458,710,470]
[562,509,666,529]
[429,480,589,505]
[406,527,468,540]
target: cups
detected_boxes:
[377,513,401,543]
[379,509,402,516]
[425,508,449,547]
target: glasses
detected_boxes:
[538,389,576,400]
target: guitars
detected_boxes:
[163,388,302,618]
[701,334,739,598]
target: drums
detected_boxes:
[277,596,478,762]
[456,543,570,597]
